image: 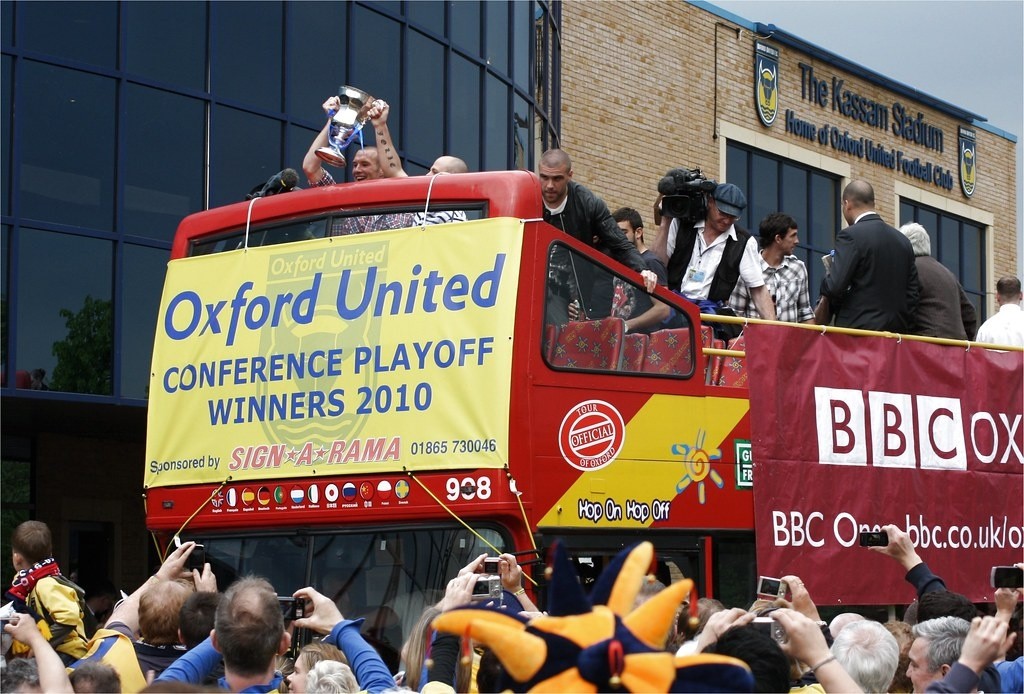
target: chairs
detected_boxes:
[543,316,749,388]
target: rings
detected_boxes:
[799,583,804,586]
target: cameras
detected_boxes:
[748,617,789,643]
[471,575,501,600]
[757,576,787,599]
[276,596,305,619]
[484,557,507,575]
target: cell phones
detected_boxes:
[860,532,889,547]
[0,618,20,634]
[990,567,1024,589]
[190,545,204,575]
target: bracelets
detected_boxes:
[808,653,836,673]
[513,589,525,596]
[625,324,629,332]
[153,574,161,582]
[816,621,827,626]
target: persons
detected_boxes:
[898,222,977,342]
[652,183,776,339]
[727,213,816,325]
[538,149,658,324]
[303,96,415,234]
[976,276,1024,348]
[31,368,49,391]
[0,520,1024,694]
[813,179,919,335]
[367,102,468,227]
[568,207,670,335]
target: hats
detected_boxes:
[715,183,747,217]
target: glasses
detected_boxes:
[717,207,740,220]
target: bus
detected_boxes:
[141,170,1023,677]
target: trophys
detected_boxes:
[314,86,376,167]
